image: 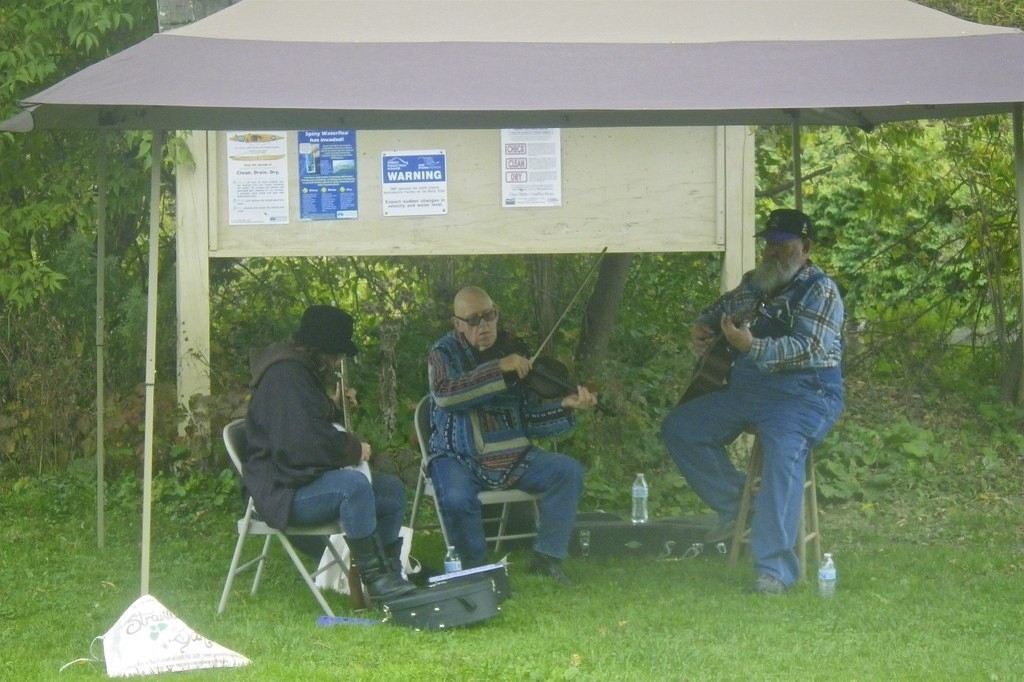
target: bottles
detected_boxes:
[817,553,836,597]
[632,473,649,523]
[444,546,461,574]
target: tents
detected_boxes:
[0,1,1024,598]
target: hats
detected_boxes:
[752,209,812,241]
[291,305,358,357]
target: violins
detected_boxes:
[509,347,617,419]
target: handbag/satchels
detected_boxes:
[315,526,421,595]
[89,595,252,678]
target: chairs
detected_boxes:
[409,394,543,553]
[217,418,349,618]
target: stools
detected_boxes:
[729,426,823,580]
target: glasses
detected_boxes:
[757,239,799,253]
[454,305,497,326]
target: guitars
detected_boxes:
[331,354,372,487]
[672,312,747,406]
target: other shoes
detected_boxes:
[528,554,571,585]
[742,574,786,594]
[704,517,752,543]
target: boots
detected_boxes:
[342,527,417,598]
[377,536,414,585]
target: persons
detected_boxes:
[242,306,421,600]
[427,285,599,581]
[662,208,845,594]
